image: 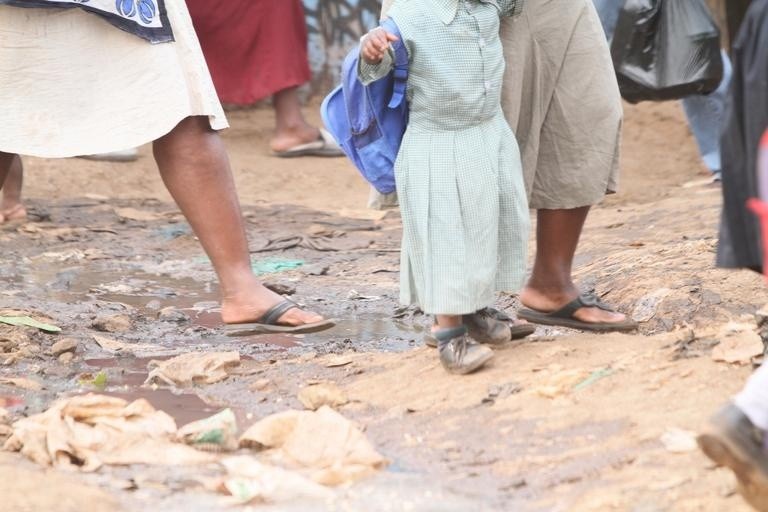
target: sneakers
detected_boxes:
[464,307,533,345]
[439,333,493,376]
[696,401,768,511]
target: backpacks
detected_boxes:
[318,18,410,193]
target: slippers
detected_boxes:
[221,300,335,336]
[517,291,638,332]
[269,126,347,160]
